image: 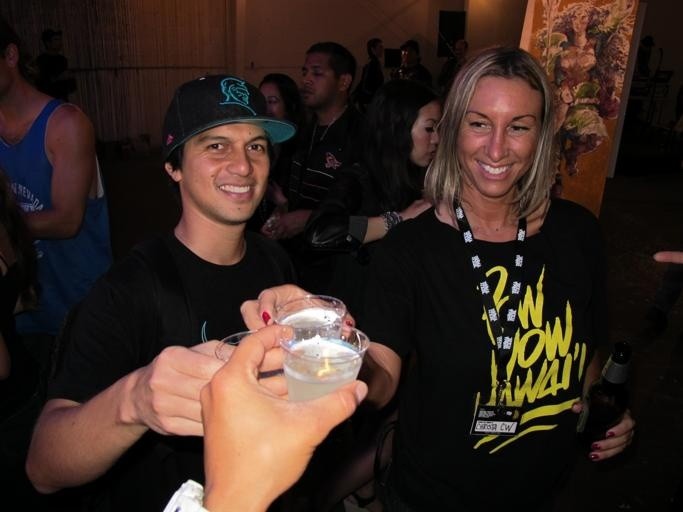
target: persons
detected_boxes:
[26,76,369,510]
[238,45,651,512]
[223,37,469,282]
[202,324,368,511]
[0,19,117,393]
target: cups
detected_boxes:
[273,295,347,347]
[280,321,369,401]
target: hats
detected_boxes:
[160,74,297,156]
